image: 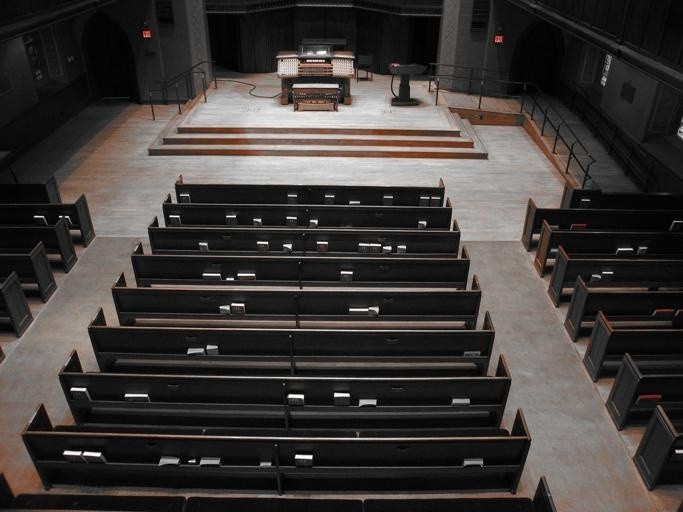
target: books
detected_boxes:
[32,215,48,226]
[69,386,91,401]
[293,453,313,467]
[634,394,662,402]
[57,215,73,226]
[62,449,87,463]
[286,393,305,406]
[197,456,222,467]
[203,344,220,355]
[185,347,206,355]
[668,449,682,463]
[332,392,350,406]
[157,455,181,467]
[259,461,272,467]
[358,398,377,408]
[569,197,682,319]
[123,392,151,402]
[82,450,107,463]
[166,194,442,316]
[450,397,470,406]
[461,457,484,468]
[187,458,196,465]
